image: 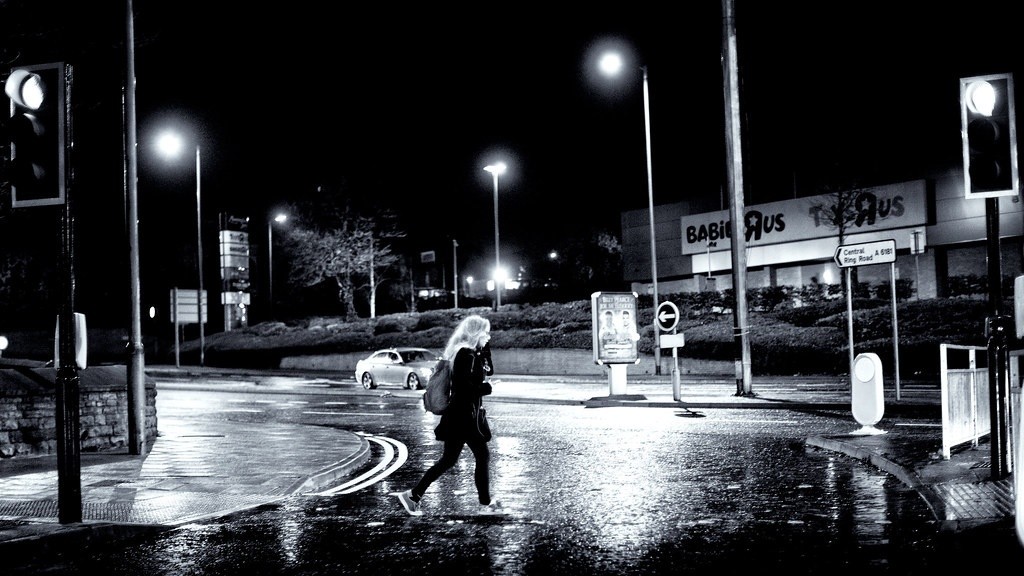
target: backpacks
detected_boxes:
[423,359,454,415]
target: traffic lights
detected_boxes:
[851,353,884,426]
[4,63,68,210]
[959,73,1020,200]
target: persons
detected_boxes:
[399,315,499,516]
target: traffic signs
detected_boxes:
[834,239,898,269]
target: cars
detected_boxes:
[355,348,443,390]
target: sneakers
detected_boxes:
[477,499,511,515]
[398,491,422,516]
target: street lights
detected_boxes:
[268,214,286,311]
[598,49,662,374]
[483,166,503,310]
[152,132,205,364]
